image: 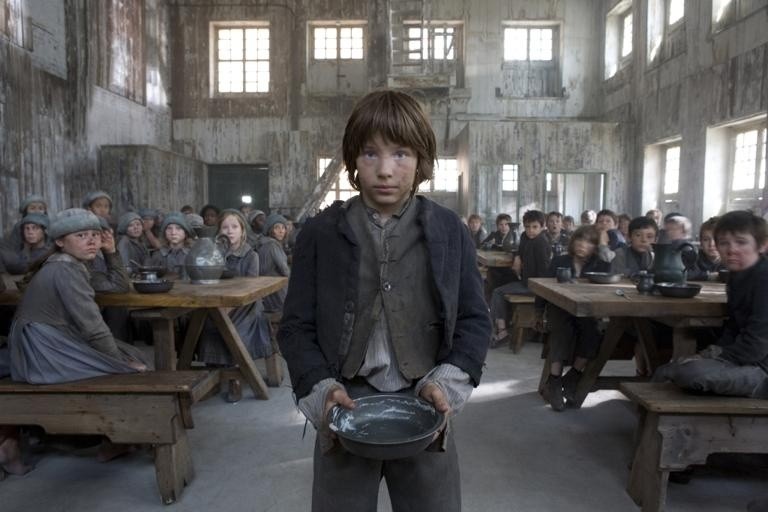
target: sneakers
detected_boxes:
[225,380,242,403]
[542,376,564,411]
[561,371,578,402]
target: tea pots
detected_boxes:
[646,239,699,285]
[183,225,232,284]
[501,222,522,252]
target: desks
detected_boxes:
[0,274,290,428]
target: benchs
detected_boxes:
[257,309,288,387]
[0,368,227,504]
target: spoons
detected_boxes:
[614,288,634,303]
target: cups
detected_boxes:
[556,266,571,284]
[631,273,655,295]
[133,271,157,280]
[717,269,729,283]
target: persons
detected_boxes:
[1,190,304,464]
[276,90,494,511]
[456,204,768,485]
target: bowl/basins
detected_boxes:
[131,280,175,294]
[583,270,625,284]
[324,392,447,463]
[655,281,703,298]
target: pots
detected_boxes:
[129,258,167,277]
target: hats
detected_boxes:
[186,212,203,228]
[140,208,155,218]
[18,194,47,213]
[19,213,48,228]
[48,208,102,242]
[116,212,143,234]
[248,209,264,224]
[262,213,289,235]
[160,213,195,246]
[82,190,112,209]
